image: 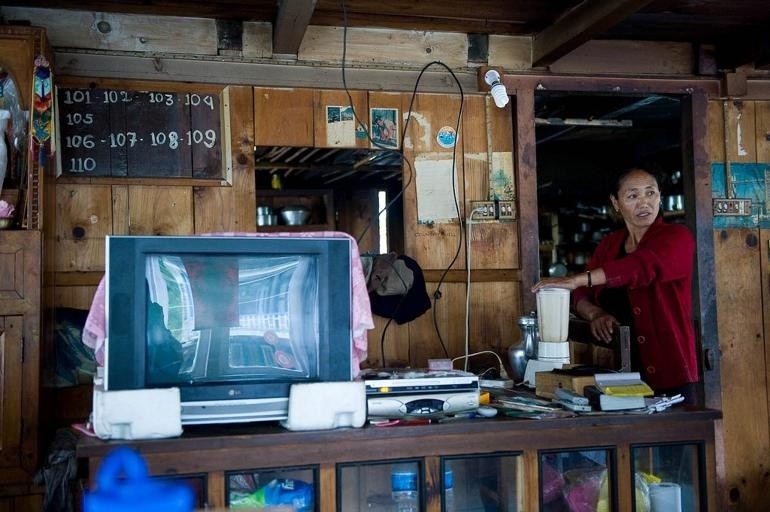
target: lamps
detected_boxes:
[484,69,509,111]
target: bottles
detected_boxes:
[444,461,458,511]
[389,462,418,511]
[1,110,10,200]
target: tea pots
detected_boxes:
[506,310,538,379]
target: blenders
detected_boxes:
[524,288,573,389]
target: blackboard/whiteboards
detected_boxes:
[52,74,233,187]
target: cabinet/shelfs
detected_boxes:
[0,23,46,511]
[71,408,725,511]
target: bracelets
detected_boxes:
[586,268,593,289]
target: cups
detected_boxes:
[562,223,607,265]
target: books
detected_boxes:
[584,371,654,411]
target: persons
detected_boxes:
[530,159,703,490]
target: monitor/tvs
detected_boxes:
[106,234,352,425]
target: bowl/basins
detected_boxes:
[256,203,278,227]
[281,209,312,226]
[664,196,686,211]
[0,215,14,229]
